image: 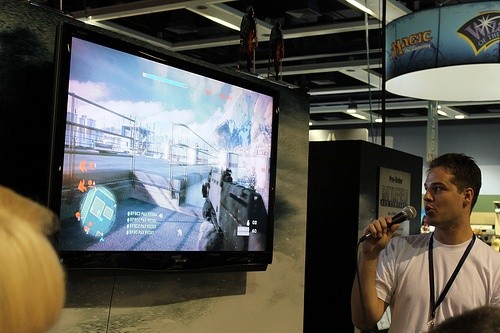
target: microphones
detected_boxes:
[359,206,417,242]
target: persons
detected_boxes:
[351,153,500,333]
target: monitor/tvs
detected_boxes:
[45,20,283,278]
